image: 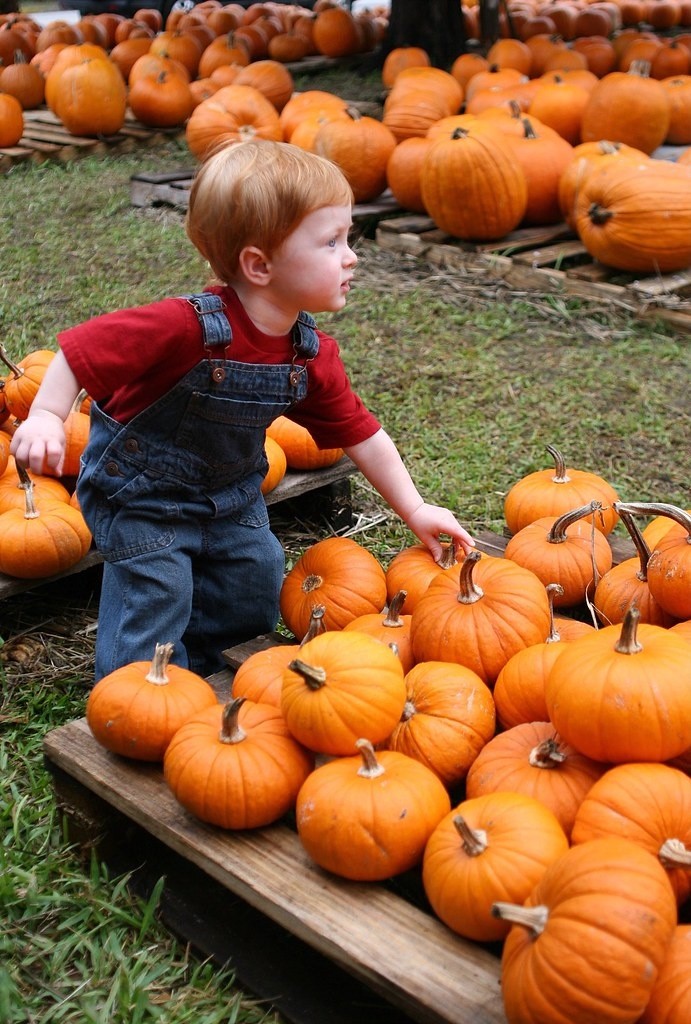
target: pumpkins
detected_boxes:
[0,0,691,270]
[85,444,691,1024]
[0,348,344,581]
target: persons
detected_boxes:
[9,140,476,681]
